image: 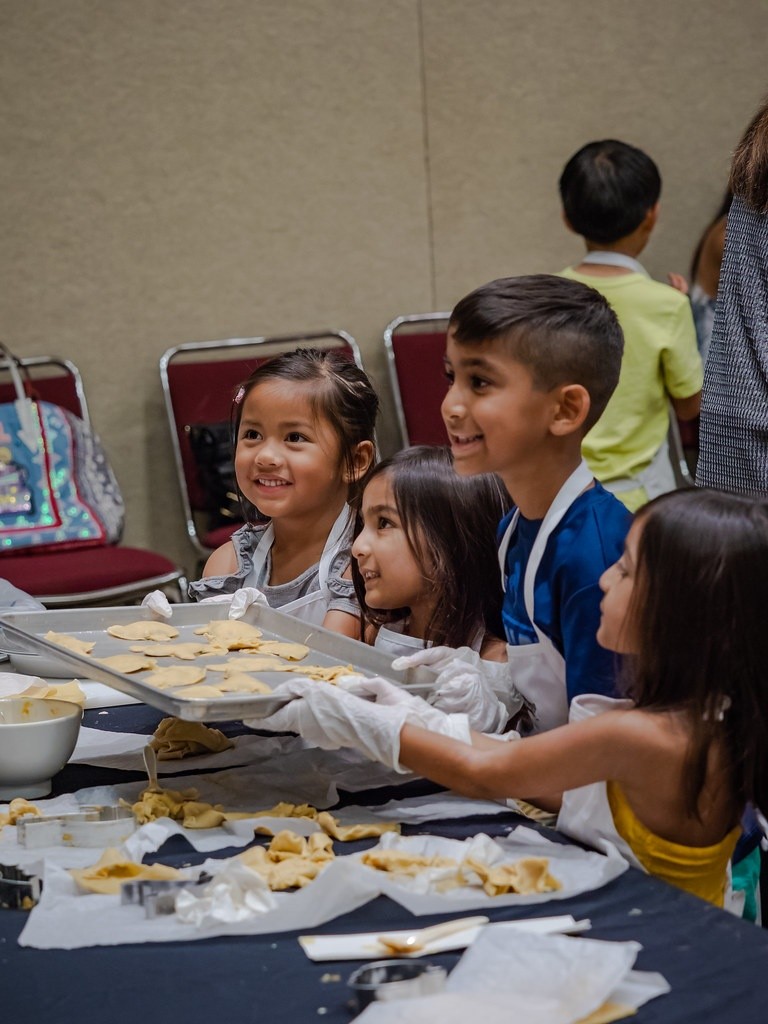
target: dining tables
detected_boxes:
[0,700,768,1024]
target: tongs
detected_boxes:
[120,879,212,919]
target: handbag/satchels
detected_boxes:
[181,423,271,532]
[0,342,126,555]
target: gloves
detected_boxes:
[335,676,473,746]
[243,678,427,774]
[391,647,525,733]
[140,587,270,621]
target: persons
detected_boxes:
[352,445,516,665]
[243,486,768,923]
[551,138,729,511]
[695,105,768,500]
[392,274,633,739]
[143,350,376,641]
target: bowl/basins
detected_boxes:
[0,697,83,802]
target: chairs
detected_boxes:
[158,328,381,555]
[383,312,452,448]
[0,355,188,604]
